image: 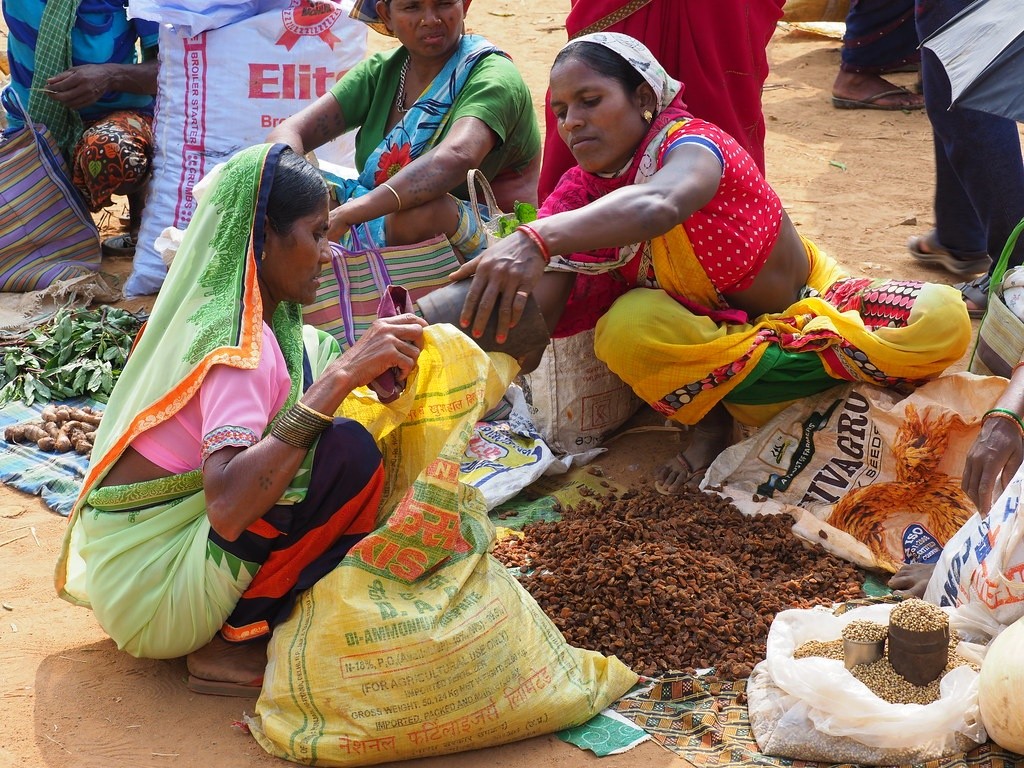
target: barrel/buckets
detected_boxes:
[888,614,949,687]
[417,269,576,357]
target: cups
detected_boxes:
[843,635,886,669]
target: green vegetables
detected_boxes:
[492,199,538,237]
[0,305,150,407]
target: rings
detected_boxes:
[516,291,528,297]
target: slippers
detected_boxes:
[909,227,992,274]
[832,84,926,110]
[655,452,716,495]
[102,233,140,256]
[948,274,994,319]
[188,669,265,697]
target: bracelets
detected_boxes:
[381,183,401,211]
[272,401,335,448]
[516,225,549,262]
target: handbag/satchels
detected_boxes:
[300,197,460,358]
[0,87,102,292]
[465,168,645,455]
[967,217,1024,379]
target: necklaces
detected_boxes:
[396,54,410,113]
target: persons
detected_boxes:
[536,0,787,211]
[52,144,431,699]
[1,0,160,245]
[445,32,973,496]
[265,0,542,261]
[828,0,1024,318]
